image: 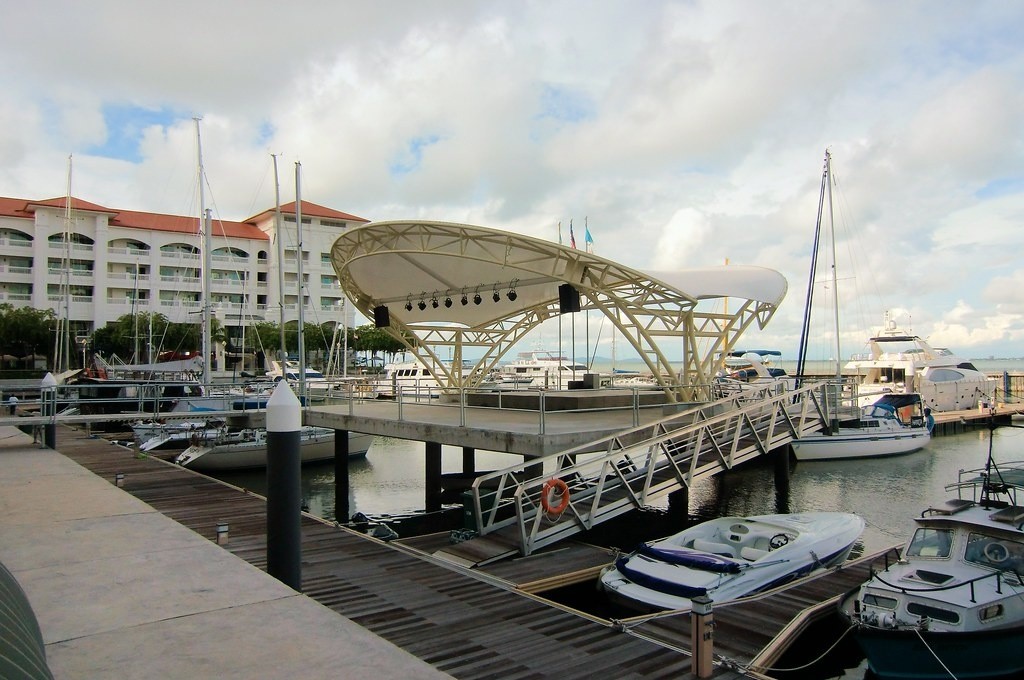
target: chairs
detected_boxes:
[740,547,771,562]
[693,539,736,559]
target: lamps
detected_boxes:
[403,277,519,311]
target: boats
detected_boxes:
[787,390,935,461]
[713,347,789,400]
[605,372,665,391]
[784,145,994,423]
[436,347,535,390]
[498,348,595,388]
[33,115,374,472]
[321,374,378,403]
[600,507,868,613]
[264,362,333,397]
[377,362,498,400]
[847,386,1024,680]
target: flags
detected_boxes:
[585,219,593,243]
[558,222,562,244]
[570,219,576,250]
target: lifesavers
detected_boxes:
[98,368,106,379]
[542,479,569,513]
[86,367,92,377]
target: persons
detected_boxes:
[8,393,19,415]
[923,408,935,431]
